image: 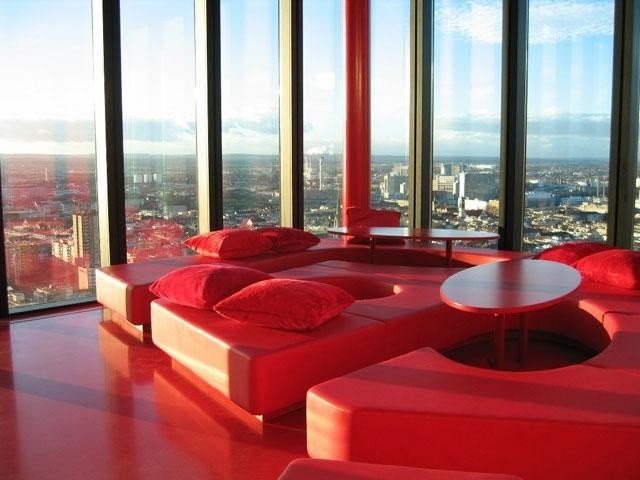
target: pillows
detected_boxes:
[255,226,321,252]
[343,205,404,245]
[182,227,273,258]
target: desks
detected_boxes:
[329,226,500,268]
